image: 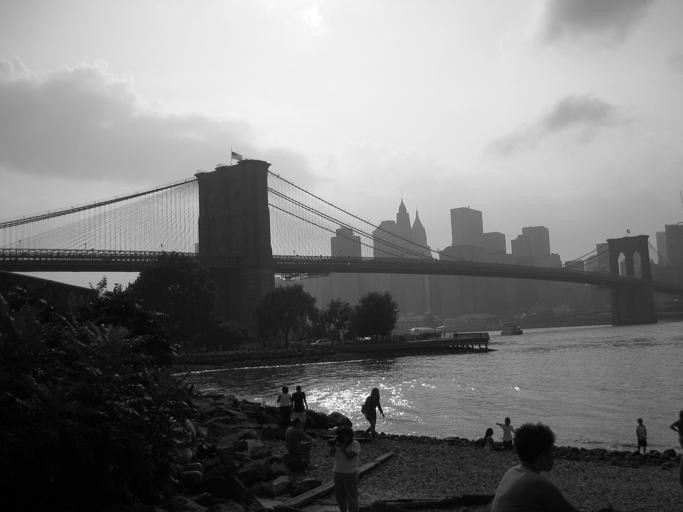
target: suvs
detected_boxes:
[308,337,333,347]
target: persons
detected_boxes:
[635,417,648,453]
[481,428,495,452]
[328,426,361,512]
[284,419,313,458]
[491,421,577,511]
[289,385,308,429]
[275,386,291,420]
[495,416,514,449]
[360,387,384,435]
[669,411,682,447]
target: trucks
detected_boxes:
[407,327,434,340]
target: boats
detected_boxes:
[452,331,490,343]
[501,324,523,335]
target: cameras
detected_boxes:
[328,438,337,446]
